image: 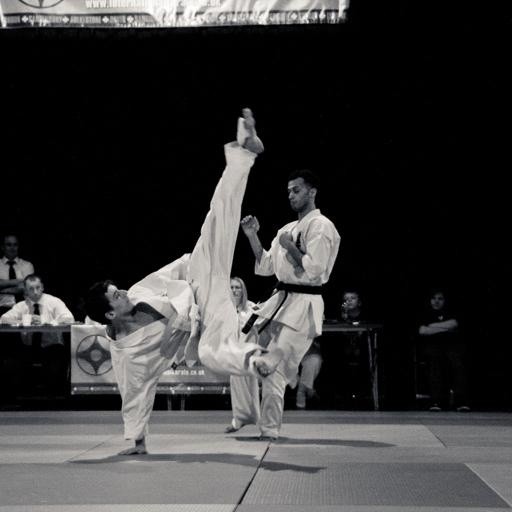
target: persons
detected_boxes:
[1,235,37,319]
[337,290,369,369]
[231,277,257,336]
[224,169,340,441]
[86,108,284,455]
[1,273,75,408]
[288,337,323,409]
[416,285,469,411]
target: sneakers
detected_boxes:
[427,402,471,413]
[296,391,307,410]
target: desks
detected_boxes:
[0,321,385,411]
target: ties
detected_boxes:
[31,302,42,348]
[8,261,25,303]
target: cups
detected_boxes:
[22,314,32,326]
